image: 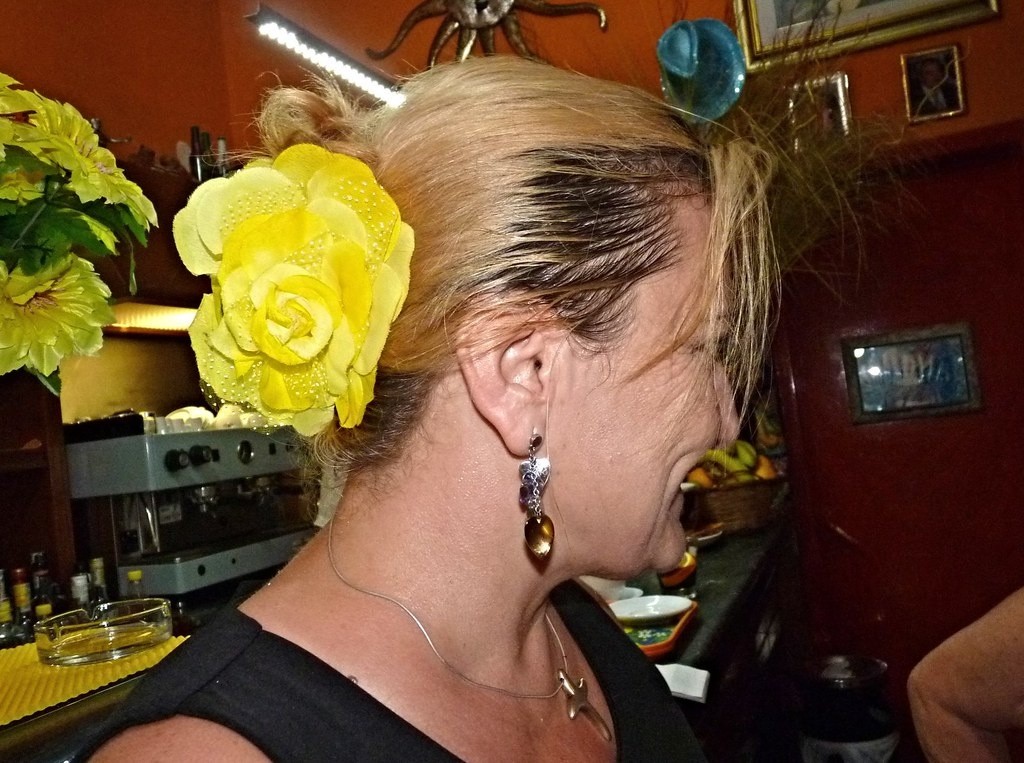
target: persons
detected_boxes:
[884,351,939,410]
[906,585,1024,763]
[910,59,960,118]
[55,54,783,763]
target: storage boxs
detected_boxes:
[696,475,783,533]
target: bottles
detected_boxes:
[0,551,107,649]
[190,126,232,182]
[127,570,144,599]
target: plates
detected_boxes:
[608,595,693,624]
[164,406,206,423]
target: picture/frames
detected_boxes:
[840,321,983,423]
[900,44,965,124]
[733,0,1000,73]
[786,72,854,154]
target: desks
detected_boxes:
[623,513,804,763]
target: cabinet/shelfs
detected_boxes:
[1,371,192,761]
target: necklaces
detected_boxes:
[327,514,612,743]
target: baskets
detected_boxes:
[684,474,789,533]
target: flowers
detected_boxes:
[172,144,417,437]
[0,70,159,398]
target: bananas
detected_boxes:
[702,440,757,483]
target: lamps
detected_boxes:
[244,3,402,103]
